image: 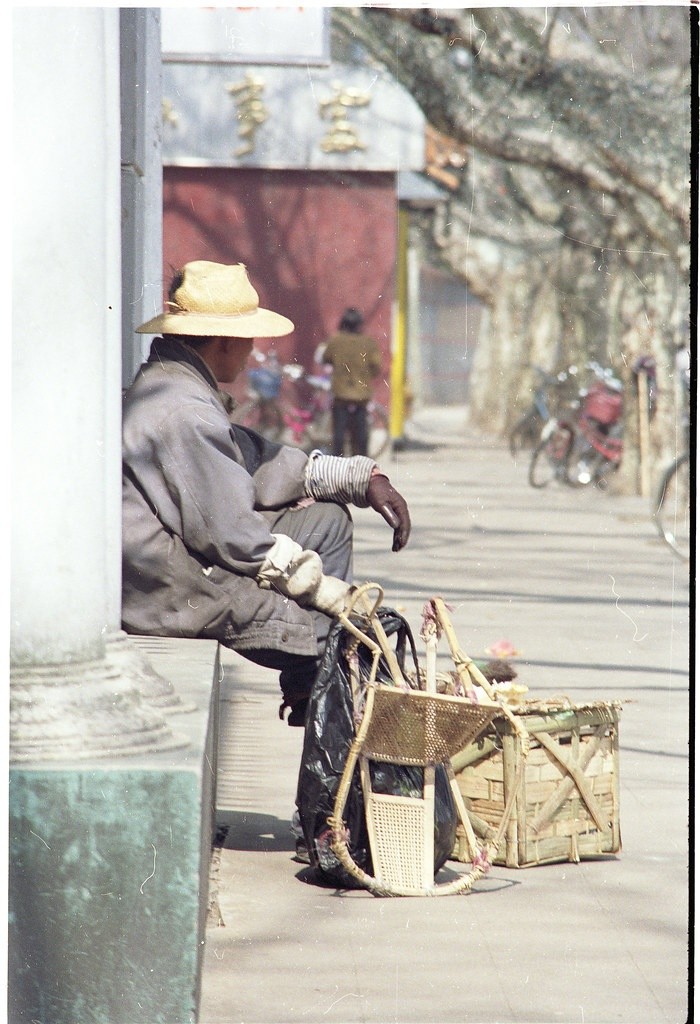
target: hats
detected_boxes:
[135,260,295,339]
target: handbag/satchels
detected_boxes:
[295,608,459,892]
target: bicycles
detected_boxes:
[510,361,690,561]
[228,347,389,462]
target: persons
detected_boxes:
[314,308,383,457]
[124,262,410,729]
[245,351,284,442]
